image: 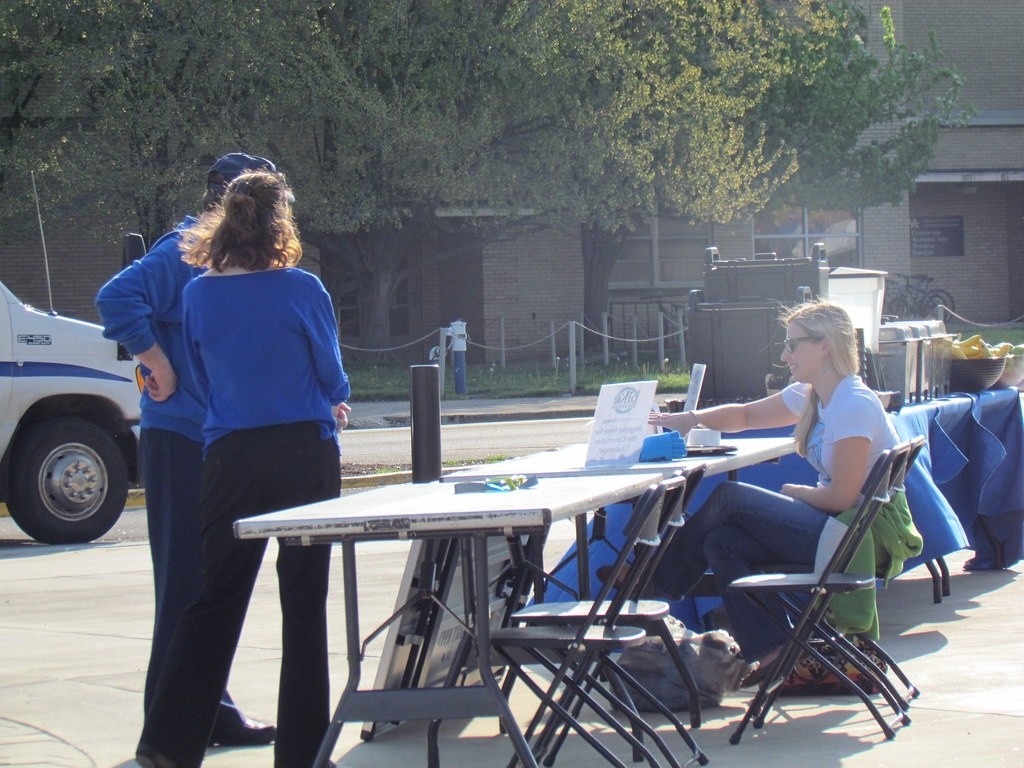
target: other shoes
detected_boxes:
[136,744,176,768]
[596,564,643,589]
[209,722,276,746]
[741,647,787,689]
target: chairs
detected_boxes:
[490,435,927,768]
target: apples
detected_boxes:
[1003,344,1024,374]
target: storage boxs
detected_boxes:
[878,333,959,406]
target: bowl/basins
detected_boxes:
[945,356,1024,394]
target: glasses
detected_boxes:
[785,336,824,353]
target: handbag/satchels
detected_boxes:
[788,631,889,696]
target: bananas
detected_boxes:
[928,333,1012,360]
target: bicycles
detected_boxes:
[880,273,955,324]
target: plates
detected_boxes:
[685,445,738,455]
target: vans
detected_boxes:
[1,279,149,547]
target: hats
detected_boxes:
[205,153,295,206]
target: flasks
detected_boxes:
[409,364,441,485]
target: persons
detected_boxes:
[596,303,902,687]
[95,153,350,768]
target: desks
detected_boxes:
[526,385,1024,616]
[229,469,672,768]
[444,437,801,738]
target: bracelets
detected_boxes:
[688,410,697,426]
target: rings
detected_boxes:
[660,414,661,418]
